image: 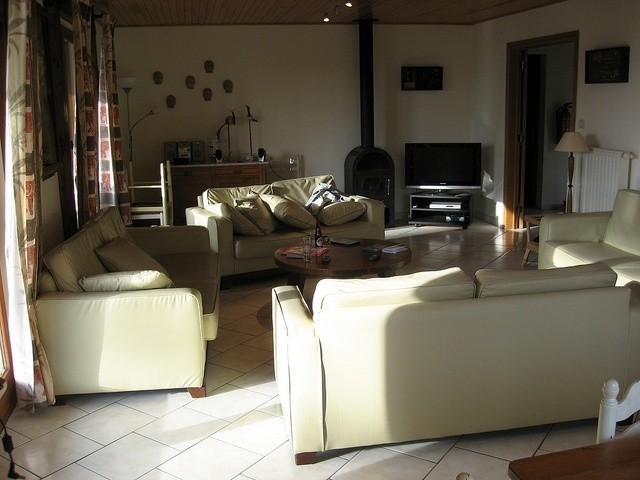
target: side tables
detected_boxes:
[518,210,544,268]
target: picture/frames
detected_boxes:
[399,65,443,91]
[584,47,631,83]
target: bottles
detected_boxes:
[315,218,323,248]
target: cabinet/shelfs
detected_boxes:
[163,162,268,227]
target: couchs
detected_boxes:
[185,174,386,291]
[538,188,640,285]
[270,265,640,464]
[34,205,222,399]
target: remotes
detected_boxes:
[321,255,330,265]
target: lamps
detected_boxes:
[117,76,162,205]
[553,132,591,214]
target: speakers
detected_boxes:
[216,149,222,163]
[257,148,264,161]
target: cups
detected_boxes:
[302,235,312,262]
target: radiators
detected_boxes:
[566,148,635,213]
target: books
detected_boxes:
[353,243,388,262]
[331,237,359,245]
[281,244,329,258]
[382,244,409,254]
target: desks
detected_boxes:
[408,191,472,231]
[507,433,640,480]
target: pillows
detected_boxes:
[234,190,278,235]
[317,200,367,227]
[93,235,169,277]
[200,202,264,238]
[258,192,318,230]
[77,270,176,292]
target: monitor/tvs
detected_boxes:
[404,144,481,196]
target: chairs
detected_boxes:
[595,378,640,445]
[129,213,163,226]
[124,158,174,229]
